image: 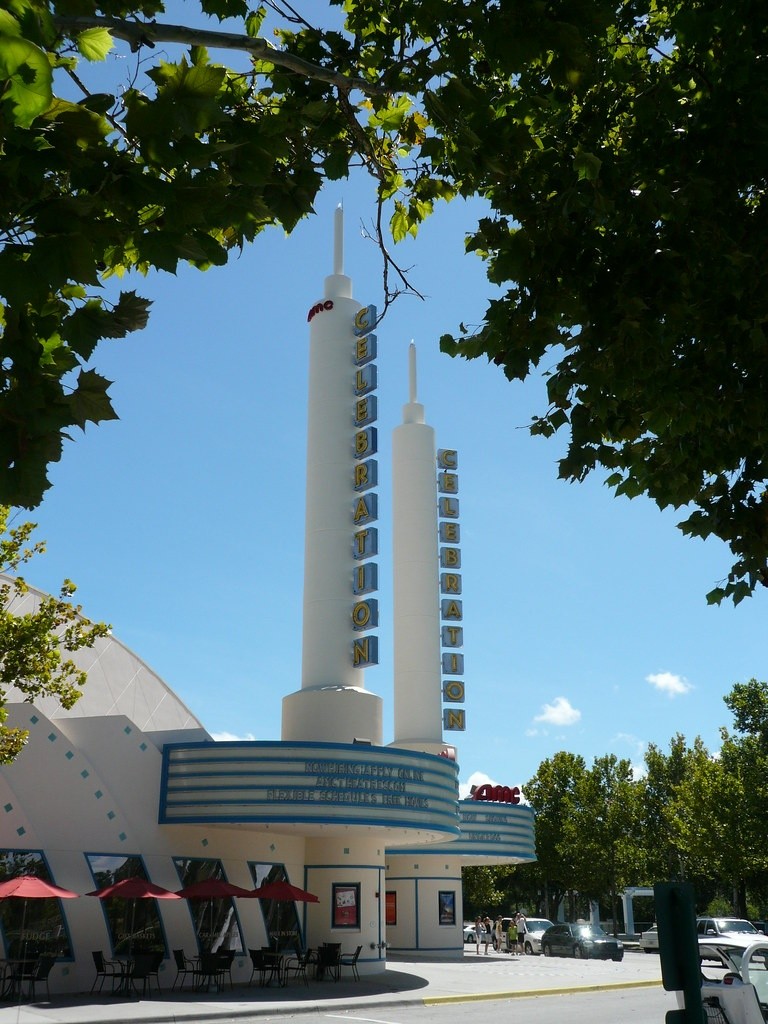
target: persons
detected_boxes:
[474,912,530,956]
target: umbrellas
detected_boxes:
[0,871,81,1001]
[85,874,182,995]
[174,877,257,991]
[245,880,321,980]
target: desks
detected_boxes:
[110,956,139,997]
[0,959,39,1003]
[193,954,222,992]
[265,953,293,988]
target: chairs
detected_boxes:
[90,942,163,999]
[248,942,363,989]
[0,946,57,1004]
[170,946,236,995]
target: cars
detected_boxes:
[463,925,495,944]
[639,926,661,953]
[540,921,624,963]
[695,916,768,968]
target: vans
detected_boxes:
[491,918,556,956]
